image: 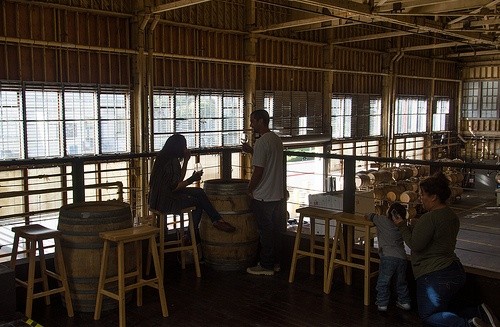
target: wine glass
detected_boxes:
[194,163,203,184]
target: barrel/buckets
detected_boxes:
[355,156,464,218]
[54,201,135,312]
[198,178,258,271]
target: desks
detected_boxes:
[201,178,261,271]
[54,201,137,312]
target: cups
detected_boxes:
[241,136,248,144]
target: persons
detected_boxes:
[368,203,412,310]
[241,110,284,276]
[392,177,500,327]
[147,134,236,264]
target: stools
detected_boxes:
[11,224,74,319]
[289,206,381,306]
[94,224,169,327]
[147,206,201,278]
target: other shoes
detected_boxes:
[396,300,411,311]
[375,301,388,312]
[183,238,203,246]
[478,302,500,327]
[246,260,282,276]
[467,316,492,327]
[213,221,236,232]
